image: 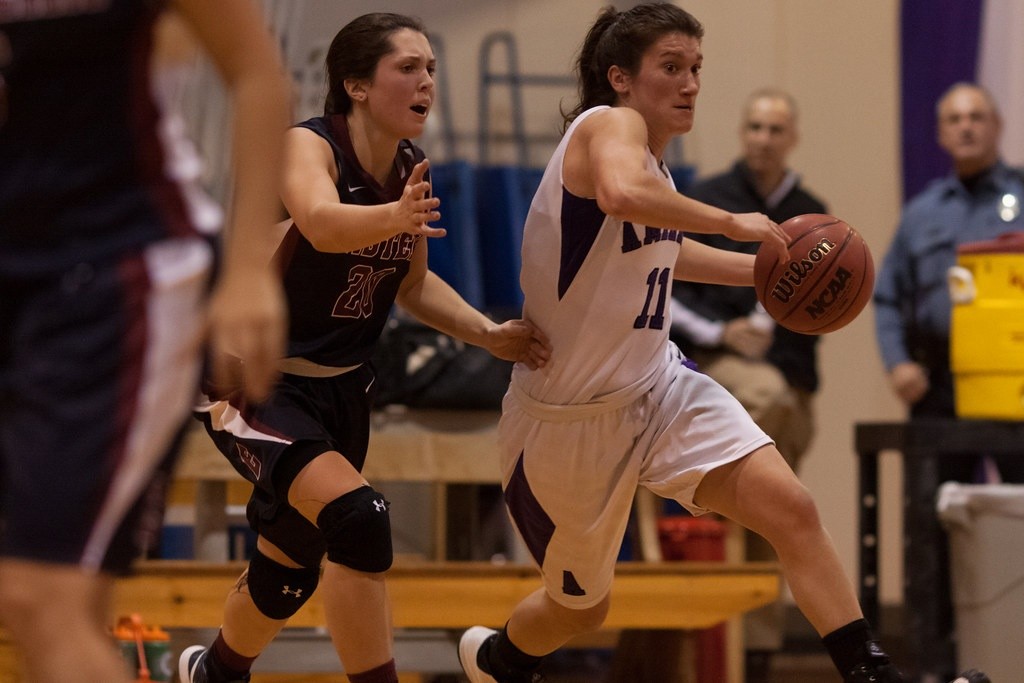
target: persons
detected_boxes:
[177,14,548,683]
[0,2,293,683]
[678,90,833,557]
[459,6,992,683]
[873,85,1024,634]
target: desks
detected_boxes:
[853,420,1024,644]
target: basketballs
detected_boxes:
[753,213,876,335]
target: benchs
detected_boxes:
[0,408,782,683]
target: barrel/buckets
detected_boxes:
[947,233,1024,423]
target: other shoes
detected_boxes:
[456,625,500,683]
[179,645,208,683]
[845,640,904,683]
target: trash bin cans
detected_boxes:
[657,517,731,683]
[936,478,1024,683]
